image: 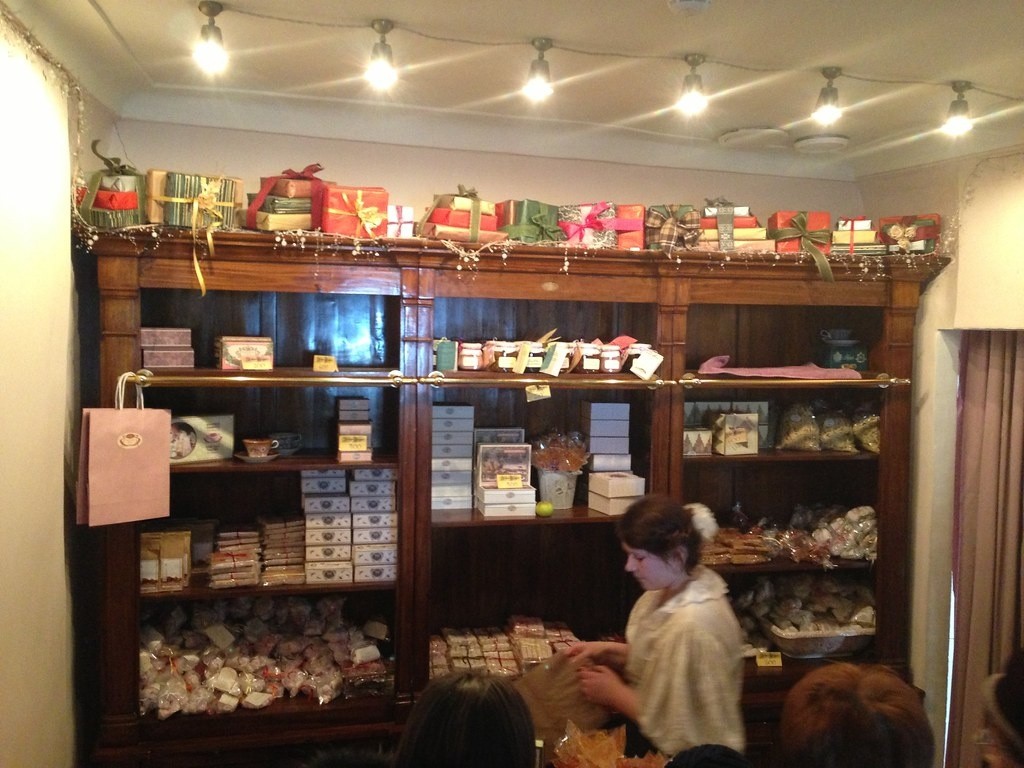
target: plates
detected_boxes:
[234,451,280,462]
[268,448,301,456]
[822,339,861,346]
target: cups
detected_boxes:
[820,328,853,339]
[242,438,279,457]
[267,433,302,449]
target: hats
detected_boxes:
[983,647,1024,748]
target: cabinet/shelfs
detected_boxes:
[77,229,418,768]
[669,251,951,768]
[414,238,678,727]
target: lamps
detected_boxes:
[524,37,552,102]
[194,0,234,71]
[813,66,842,121]
[368,19,397,86]
[942,80,972,135]
[681,55,708,115]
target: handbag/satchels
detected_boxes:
[76,371,170,526]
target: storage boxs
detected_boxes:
[80,139,941,254]
[816,344,868,370]
[683,398,778,455]
[139,521,215,594]
[301,395,646,585]
[139,324,274,372]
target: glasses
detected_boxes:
[971,729,995,749]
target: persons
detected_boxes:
[563,496,746,757]
[312,669,536,768]
[665,743,752,768]
[980,648,1024,768]
[780,663,935,768]
